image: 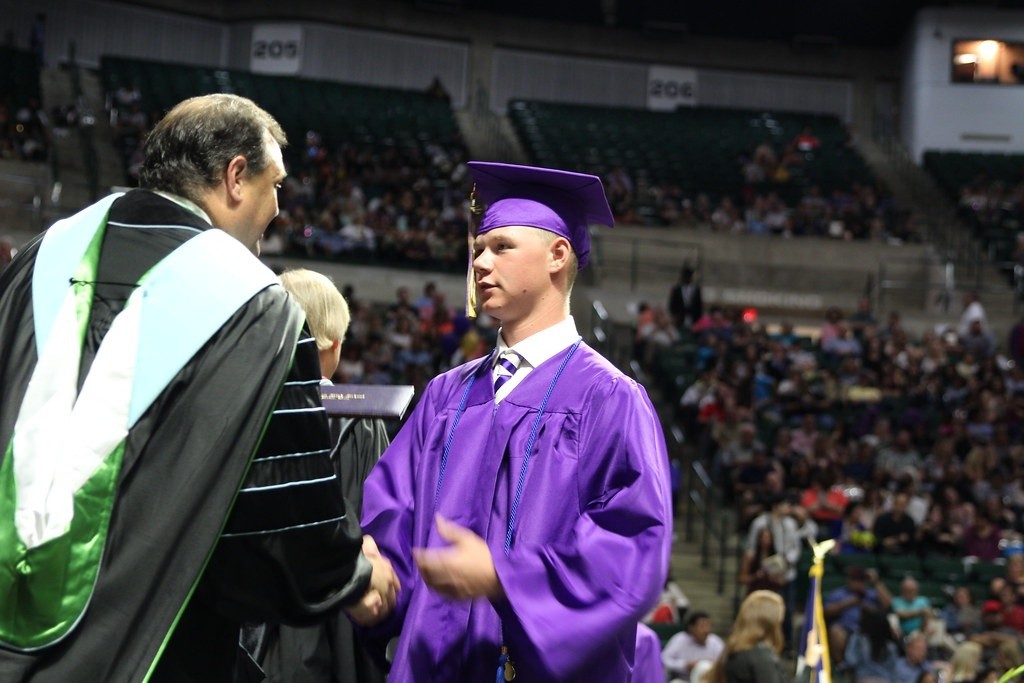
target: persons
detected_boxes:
[0,93,401,683]
[332,284,500,387]
[0,12,479,268]
[590,126,923,245]
[959,171,1024,298]
[240,270,390,683]
[340,160,672,683]
[638,257,1024,683]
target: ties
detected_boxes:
[493,351,520,402]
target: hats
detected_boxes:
[465,159,619,321]
